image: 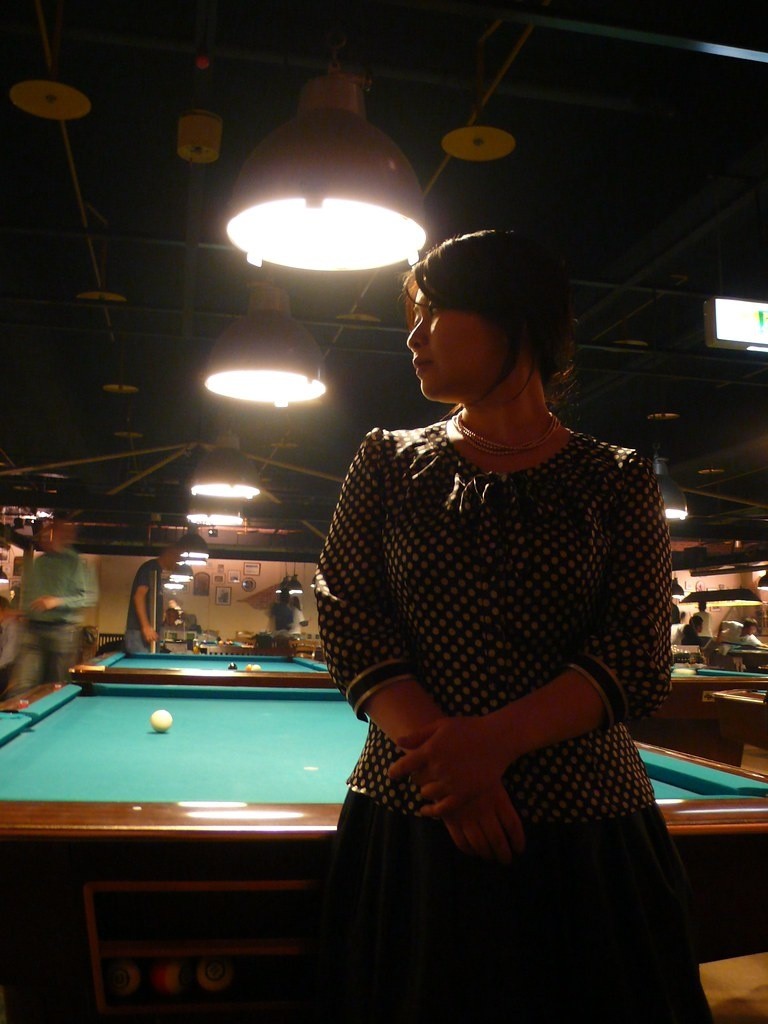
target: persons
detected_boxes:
[315,229,672,1024]
[681,602,768,658]
[124,536,202,654]
[0,518,99,698]
[265,592,305,639]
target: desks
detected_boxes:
[0,678,768,1024]
[67,652,335,687]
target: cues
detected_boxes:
[720,641,768,648]
[151,569,158,653]
[688,611,704,654]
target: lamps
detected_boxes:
[275,562,299,592]
[187,419,261,506]
[219,40,431,290]
[198,276,333,424]
[185,500,248,531]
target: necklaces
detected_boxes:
[452,411,561,456]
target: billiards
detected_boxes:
[217,640,234,646]
[149,708,173,733]
[245,663,262,671]
[227,662,238,670]
